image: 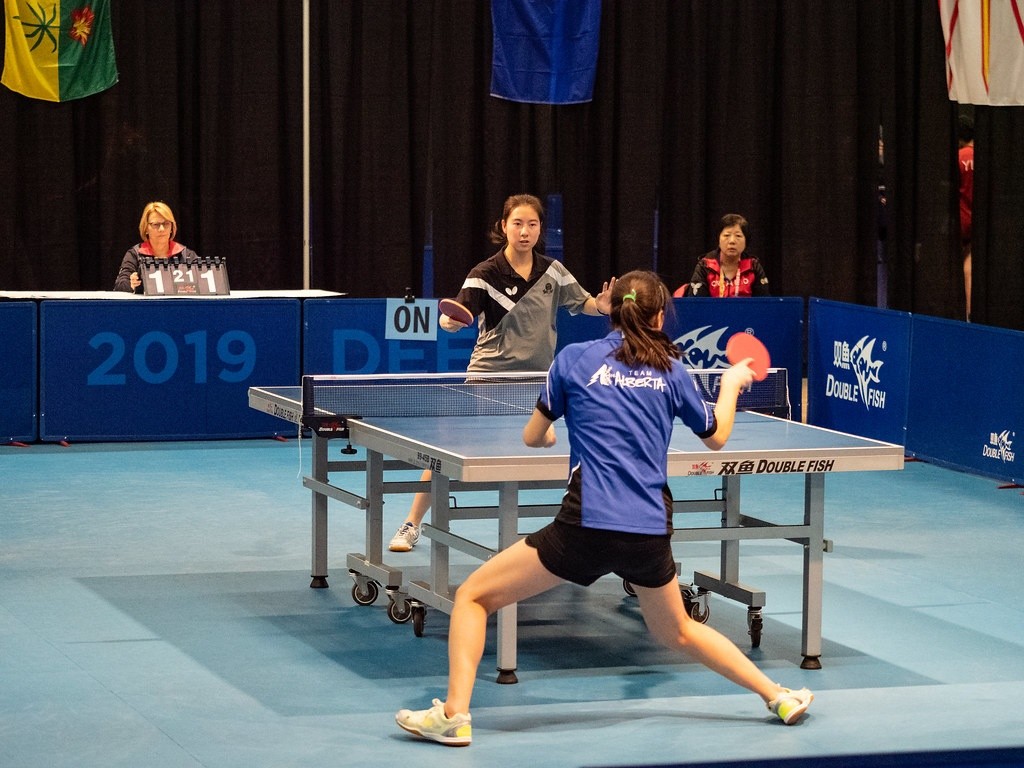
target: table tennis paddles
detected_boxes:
[725,332,770,394]
[439,298,474,333]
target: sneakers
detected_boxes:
[396,698,472,746]
[766,683,814,724]
[389,522,420,550]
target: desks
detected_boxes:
[246,368,904,686]
[0,285,348,303]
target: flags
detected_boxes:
[490,0,604,102]
[939,0,1023,106]
[2,0,120,102]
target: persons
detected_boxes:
[116,201,200,293]
[387,194,620,553]
[395,274,813,746]
[684,216,770,298]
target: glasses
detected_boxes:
[148,220,171,229]
